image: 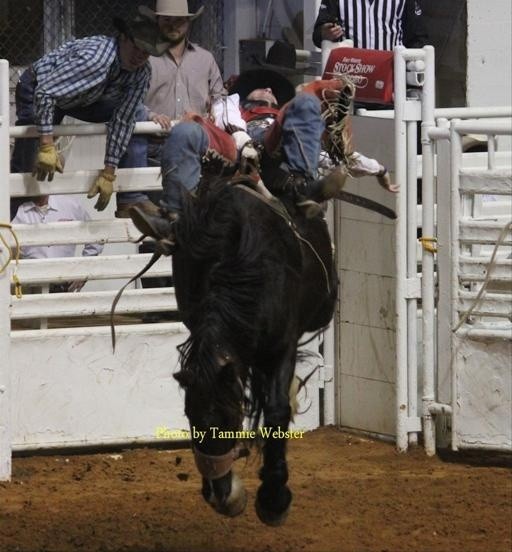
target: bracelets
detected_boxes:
[377,165,388,178]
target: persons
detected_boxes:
[10,5,183,222]
[130,68,400,256]
[11,196,104,293]
[135,0,227,205]
[312,1,428,115]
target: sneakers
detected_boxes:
[115,199,160,218]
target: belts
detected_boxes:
[336,190,396,219]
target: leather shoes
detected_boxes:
[129,204,181,255]
[283,165,347,219]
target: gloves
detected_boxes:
[240,144,259,174]
[87,170,116,211]
[31,144,63,181]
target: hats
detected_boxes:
[113,17,170,56]
[234,69,295,106]
[138,0,204,20]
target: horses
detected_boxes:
[169,159,341,527]
